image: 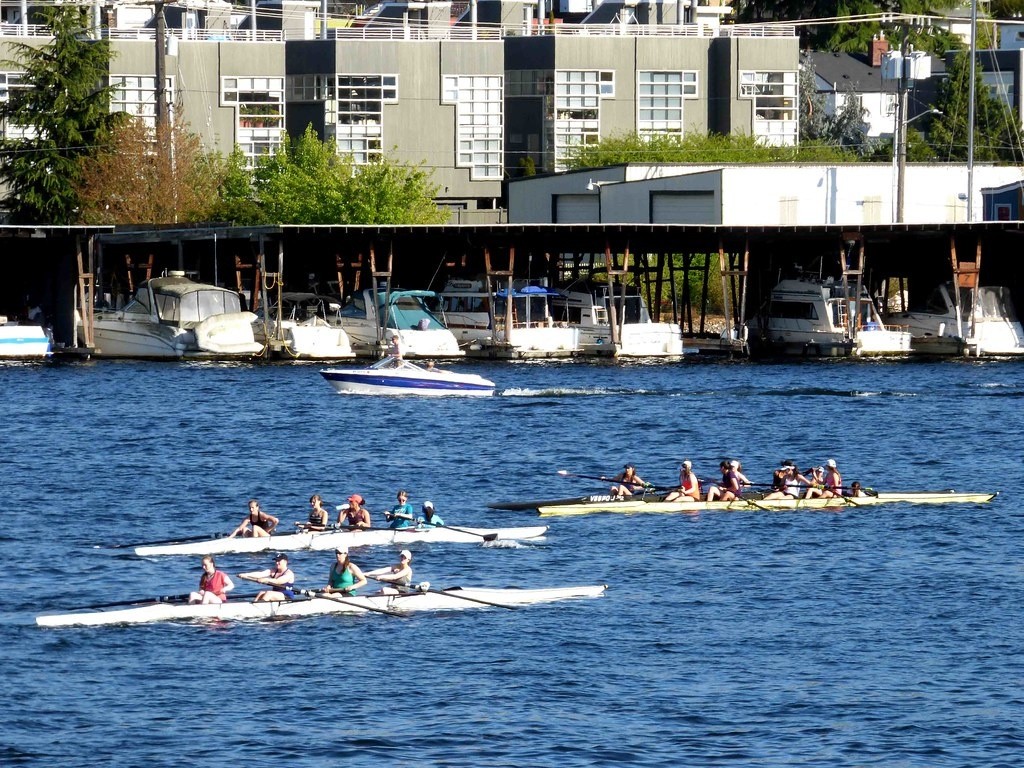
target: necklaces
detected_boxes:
[762,460,814,500]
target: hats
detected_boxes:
[682,461,691,468]
[730,460,739,469]
[781,465,795,470]
[398,550,411,560]
[623,463,635,469]
[823,459,836,467]
[335,545,349,554]
[347,495,363,504]
[816,466,824,472]
[423,501,434,510]
[272,553,288,561]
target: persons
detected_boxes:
[294,495,328,532]
[388,335,403,368]
[847,483,869,497]
[770,459,812,489]
[806,458,841,499]
[730,460,753,487]
[385,491,414,528]
[229,499,279,537]
[661,460,701,502]
[707,461,743,501]
[239,553,294,602]
[322,544,366,597]
[804,466,825,491]
[364,550,412,595]
[338,494,372,532]
[425,360,435,371]
[188,556,235,605]
[601,462,651,496]
[418,501,444,528]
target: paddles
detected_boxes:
[707,476,769,512]
[743,481,873,491]
[364,576,521,610]
[830,488,859,506]
[112,532,232,548]
[227,588,345,600]
[383,511,498,541]
[66,593,190,611]
[557,469,680,492]
[238,575,411,618]
[297,522,423,533]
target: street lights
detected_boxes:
[891,109,943,222]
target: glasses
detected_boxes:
[336,551,346,554]
[399,500,407,503]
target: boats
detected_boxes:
[76,229,272,363]
[0,315,51,362]
[880,273,1024,362]
[134,525,551,559]
[34,584,610,630]
[733,254,917,363]
[236,250,686,364]
[536,490,1001,516]
[317,353,498,398]
